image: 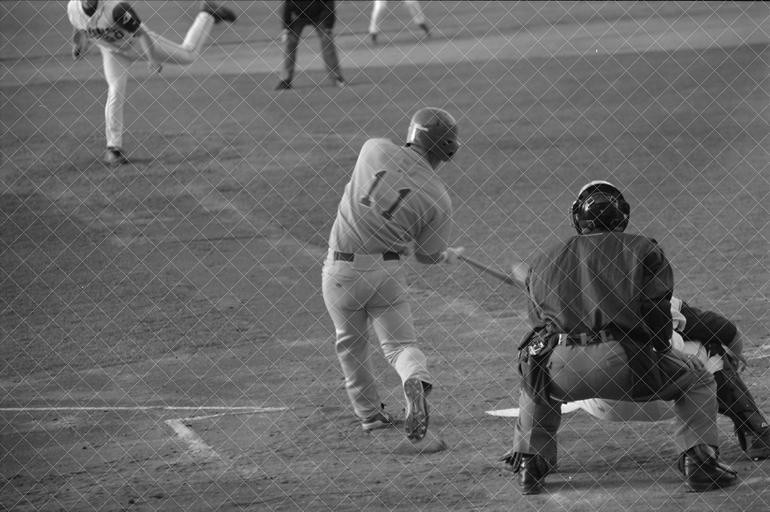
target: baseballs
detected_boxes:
[514,263,530,282]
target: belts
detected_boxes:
[332,252,399,261]
[558,332,613,345]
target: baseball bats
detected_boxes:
[459,253,525,288]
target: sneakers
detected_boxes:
[511,446,556,497]
[202,0,237,25]
[105,148,126,166]
[361,410,392,432]
[739,425,770,460]
[686,449,736,488]
[404,377,429,444]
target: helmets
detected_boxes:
[406,107,460,162]
[573,181,629,235]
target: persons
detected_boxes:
[322,107,461,443]
[67,1,238,166]
[572,295,770,460]
[506,179,739,491]
[273,0,345,90]
[368,0,431,45]
